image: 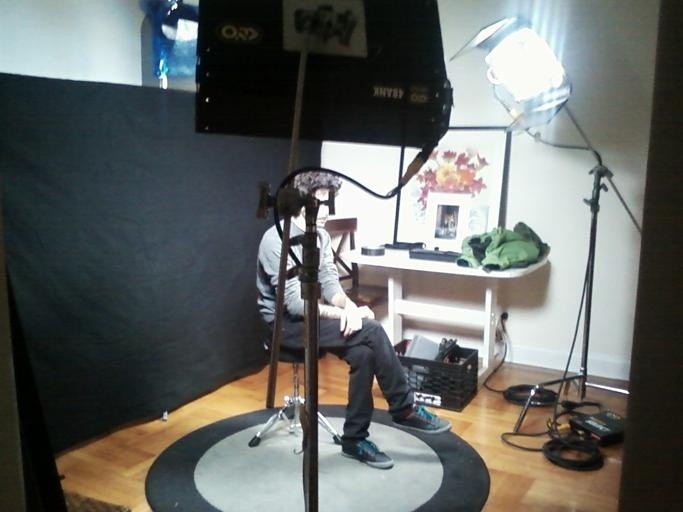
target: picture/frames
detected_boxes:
[394,126,512,256]
[422,192,472,254]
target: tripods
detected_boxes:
[248,363,344,448]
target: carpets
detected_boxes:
[144,404,490,512]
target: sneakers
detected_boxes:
[340,441,393,469]
[392,408,451,435]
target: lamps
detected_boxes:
[449,15,641,417]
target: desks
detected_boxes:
[342,245,547,391]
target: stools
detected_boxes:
[248,342,341,447]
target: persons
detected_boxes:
[254,170,452,469]
